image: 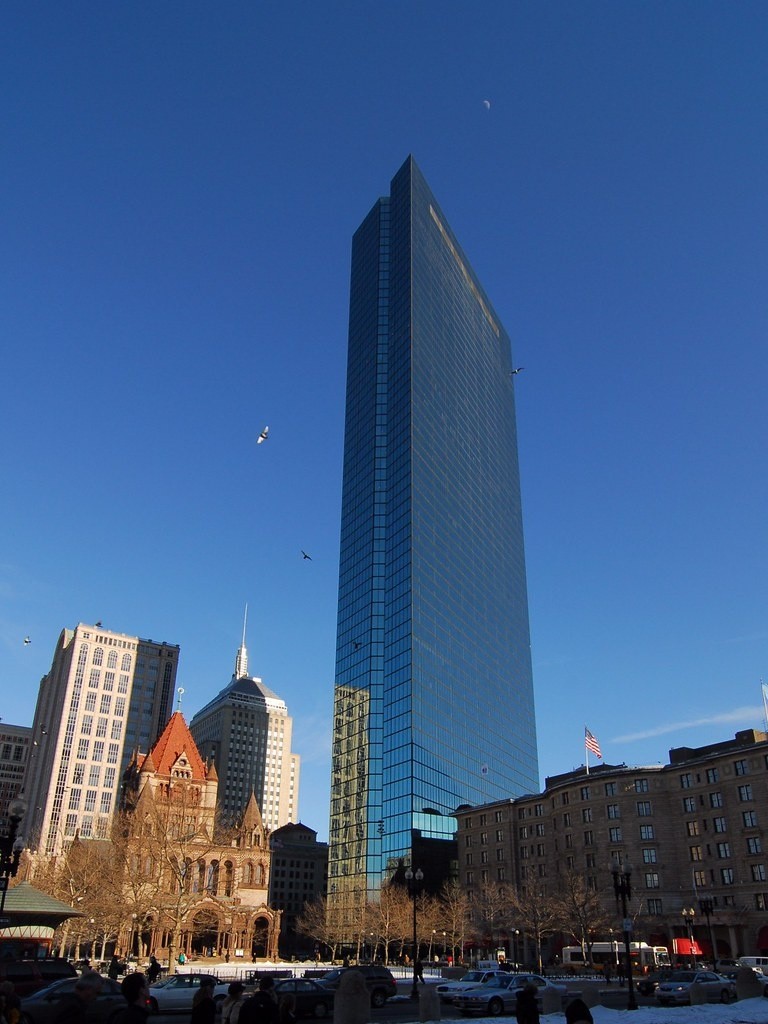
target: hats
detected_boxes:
[228,983,246,994]
[200,977,216,987]
[565,999,593,1024]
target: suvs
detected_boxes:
[0,955,79,998]
[310,965,398,1009]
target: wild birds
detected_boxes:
[23,636,32,646]
[300,550,313,561]
[510,368,524,374]
[351,640,362,649]
[257,425,269,444]
[30,724,48,758]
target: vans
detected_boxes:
[738,956,768,976]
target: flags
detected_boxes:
[585,729,601,758]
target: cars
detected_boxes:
[274,978,337,1020]
[19,976,129,1024]
[142,973,230,1016]
[654,970,736,1007]
[637,958,768,997]
[452,973,569,1017]
[435,970,512,1005]
[241,984,261,999]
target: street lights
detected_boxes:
[681,906,696,970]
[515,929,519,961]
[698,892,719,973]
[443,931,446,961]
[404,866,425,997]
[432,929,436,963]
[607,851,638,1010]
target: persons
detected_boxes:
[416,956,427,985]
[0,981,28,1024]
[581,960,594,969]
[178,952,185,965]
[146,956,160,983]
[50,983,97,1024]
[472,961,477,970]
[565,999,594,1024]
[221,983,246,1024]
[82,958,92,975]
[237,975,281,1024]
[604,960,612,984]
[273,953,276,963]
[108,955,123,981]
[616,960,626,984]
[331,952,466,968]
[515,984,542,1024]
[278,991,298,1024]
[190,978,218,1024]
[225,953,230,963]
[107,973,151,1024]
[251,953,257,963]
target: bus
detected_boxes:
[562,941,672,979]
